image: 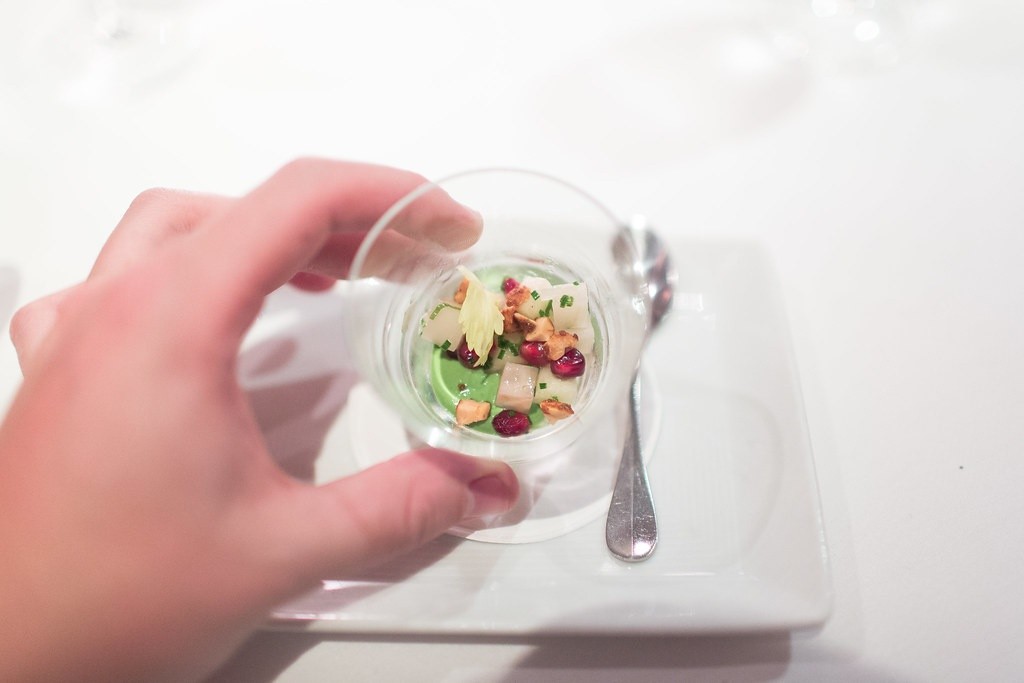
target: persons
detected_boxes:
[1,154,520,683]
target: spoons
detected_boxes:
[604,222,675,563]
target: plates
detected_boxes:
[204,216,833,639]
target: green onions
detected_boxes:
[430,281,578,390]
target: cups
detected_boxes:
[342,168,646,468]
